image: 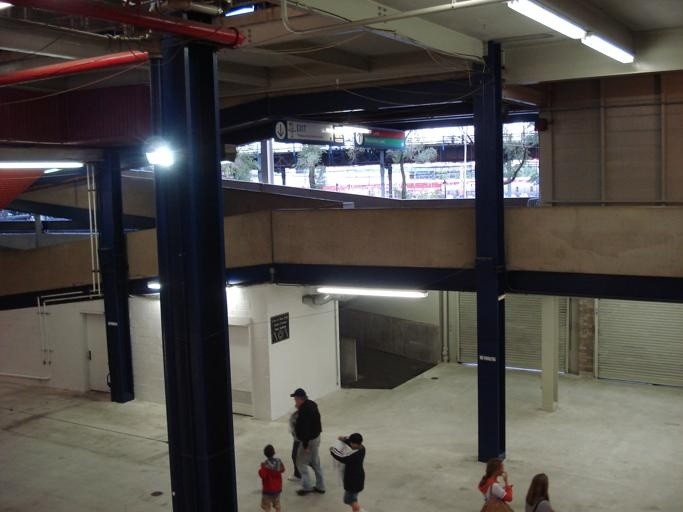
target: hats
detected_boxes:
[291,389,305,396]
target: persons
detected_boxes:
[523,473,554,512]
[329,431,367,512]
[478,459,513,512]
[286,402,301,481]
[289,387,326,495]
[256,444,284,512]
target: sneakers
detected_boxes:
[297,486,325,495]
[289,475,301,480]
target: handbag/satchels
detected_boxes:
[481,496,513,512]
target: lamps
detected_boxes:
[508,0,639,67]
